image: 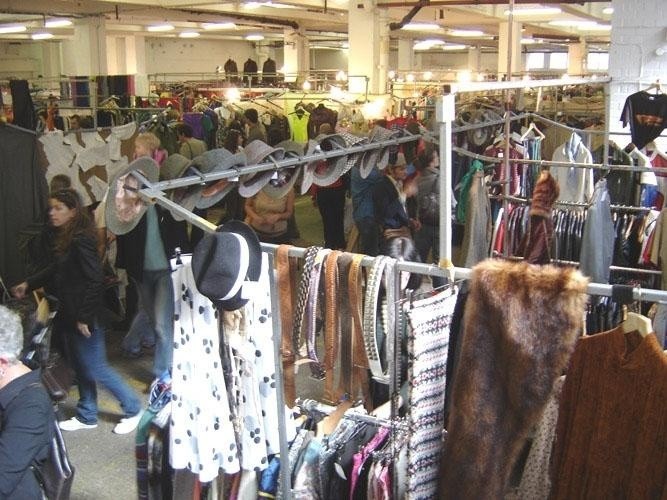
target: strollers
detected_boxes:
[0,287,66,414]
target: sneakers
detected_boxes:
[113,409,145,434]
[119,340,156,358]
[58,416,98,431]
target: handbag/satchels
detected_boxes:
[419,174,457,228]
[37,344,78,401]
[2,382,76,500]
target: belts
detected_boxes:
[292,245,400,436]
[276,243,297,410]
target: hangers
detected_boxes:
[252,397,409,494]
[624,75,667,108]
[485,194,658,246]
[220,99,362,126]
[574,284,666,371]
[489,115,666,182]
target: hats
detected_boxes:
[191,219,263,312]
[104,155,161,236]
[262,139,304,200]
[195,147,248,210]
[158,152,209,222]
[457,110,502,150]
[340,122,420,180]
[301,134,348,195]
[237,138,286,199]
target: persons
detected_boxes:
[0,303,54,499]
[131,129,166,167]
[216,129,244,228]
[44,98,64,135]
[242,110,267,151]
[67,116,81,132]
[240,182,297,248]
[117,199,189,359]
[16,188,149,436]
[312,116,491,267]
[175,123,208,249]
